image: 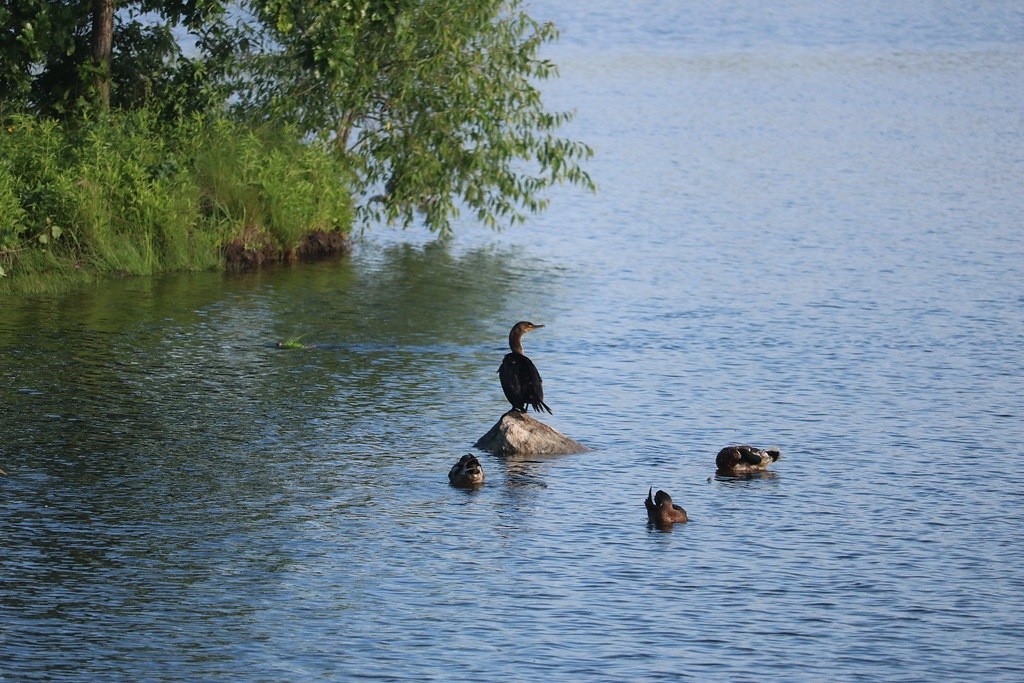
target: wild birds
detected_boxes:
[448,453,484,485]
[716,446,781,471]
[644,486,687,523]
[277,333,314,348]
[496,321,553,416]
